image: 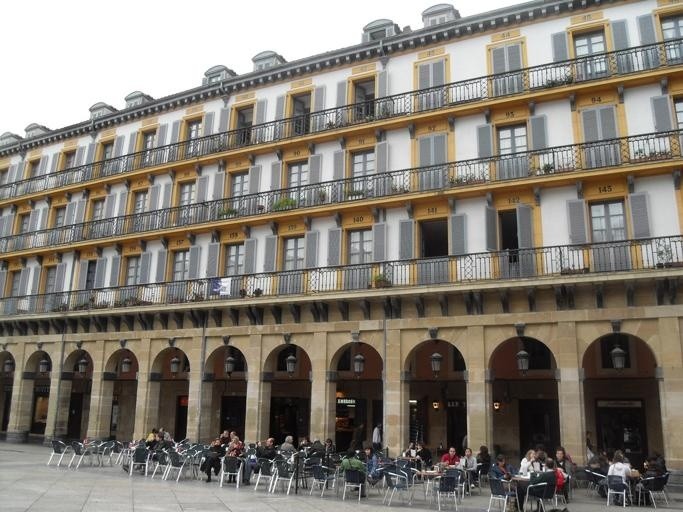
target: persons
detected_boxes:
[585,430,670,506]
[405,441,491,494]
[256,425,383,498]
[201,430,254,486]
[124,425,177,473]
[487,444,574,511]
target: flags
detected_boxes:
[209,277,231,295]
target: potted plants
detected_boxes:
[374,273,391,288]
[656,243,683,269]
[238,287,263,297]
